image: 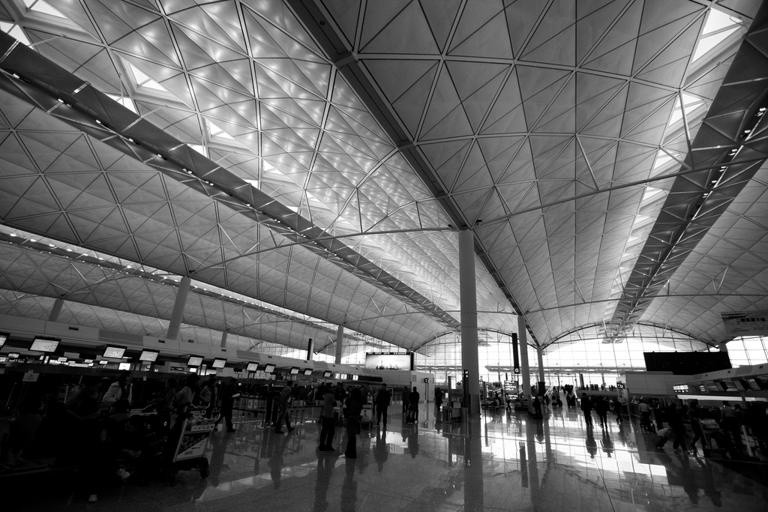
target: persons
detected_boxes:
[434,411,445,436]
[264,379,391,458]
[402,386,419,424]
[659,459,760,511]
[434,386,443,411]
[2,362,242,511]
[532,380,767,461]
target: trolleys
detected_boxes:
[167,406,224,484]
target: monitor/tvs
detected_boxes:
[28,336,60,353]
[713,378,762,391]
[139,349,159,363]
[0,330,11,349]
[102,343,127,359]
[187,355,360,382]
